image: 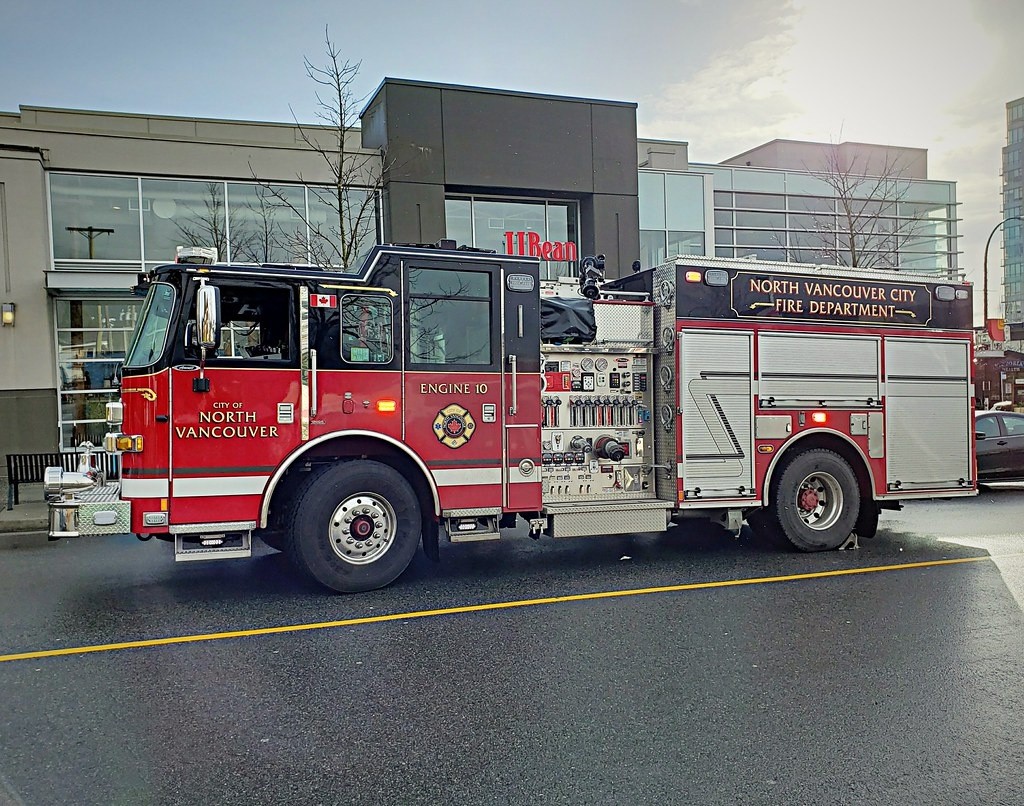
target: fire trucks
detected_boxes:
[42,239,982,598]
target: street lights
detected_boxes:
[984,216,1024,410]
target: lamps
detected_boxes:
[2,302,15,327]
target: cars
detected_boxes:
[976,408,1024,482]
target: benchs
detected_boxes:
[5,451,119,511]
